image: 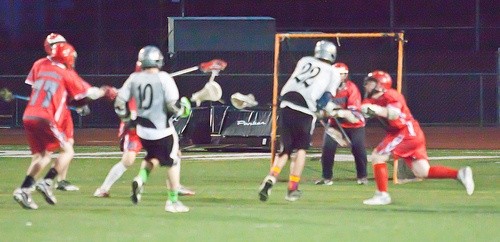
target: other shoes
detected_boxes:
[357,177,368,185]
[323,178,333,186]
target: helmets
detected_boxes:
[51,43,77,66]
[365,70,392,92]
[135,60,143,71]
[138,46,164,69]
[314,40,337,63]
[331,62,349,74]
[44,33,67,55]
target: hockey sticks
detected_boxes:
[333,107,375,115]
[333,117,357,144]
[189,81,222,107]
[116,59,227,94]
[231,93,280,111]
[0,88,90,116]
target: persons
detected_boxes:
[114,46,192,213]
[258,40,342,202]
[94,61,195,198]
[347,70,474,205]
[12,43,109,210]
[315,63,368,186]
[24,33,79,191]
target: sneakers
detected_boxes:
[457,166,475,196]
[36,178,56,205]
[258,175,276,202]
[177,184,195,196]
[54,180,80,191]
[131,176,143,204]
[164,199,189,212]
[285,188,302,201]
[93,188,110,197]
[12,187,39,210]
[362,190,391,205]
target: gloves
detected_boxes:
[321,101,340,118]
[79,105,91,116]
[102,85,117,100]
[124,119,137,136]
[361,103,382,118]
[180,96,191,118]
